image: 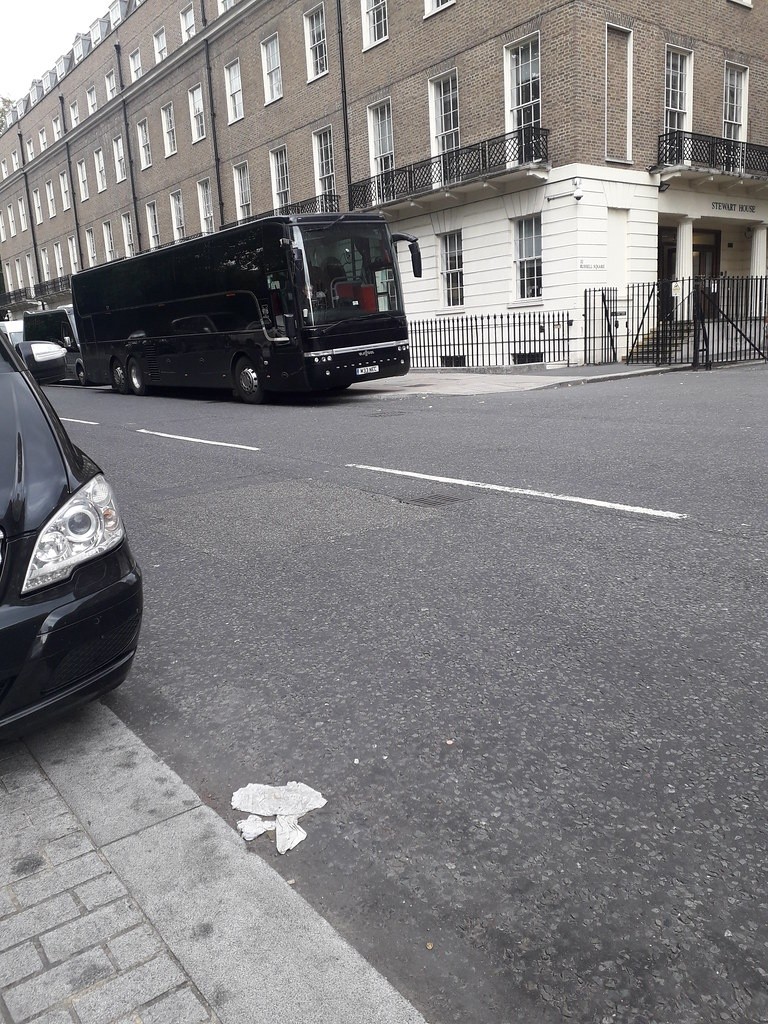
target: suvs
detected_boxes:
[158,312,246,354]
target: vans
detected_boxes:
[23,304,88,386]
[0,321,22,351]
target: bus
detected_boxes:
[71,210,421,404]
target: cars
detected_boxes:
[0,331,147,742]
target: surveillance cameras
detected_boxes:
[574,189,584,201]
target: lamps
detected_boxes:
[660,182,670,193]
[646,165,663,174]
[572,178,583,200]
